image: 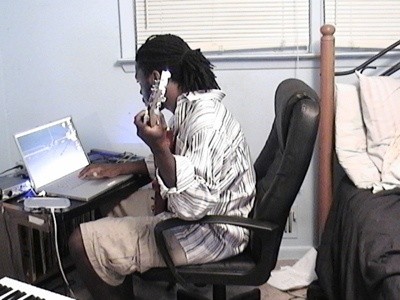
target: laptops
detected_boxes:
[12,114,133,203]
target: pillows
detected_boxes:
[357,71,399,186]
[333,82,384,190]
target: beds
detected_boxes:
[309,26,400,300]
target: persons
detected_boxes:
[69,33,257,299]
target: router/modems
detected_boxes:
[23,196,70,214]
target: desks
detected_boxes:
[0,149,154,292]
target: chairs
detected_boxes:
[135,79,321,300]
